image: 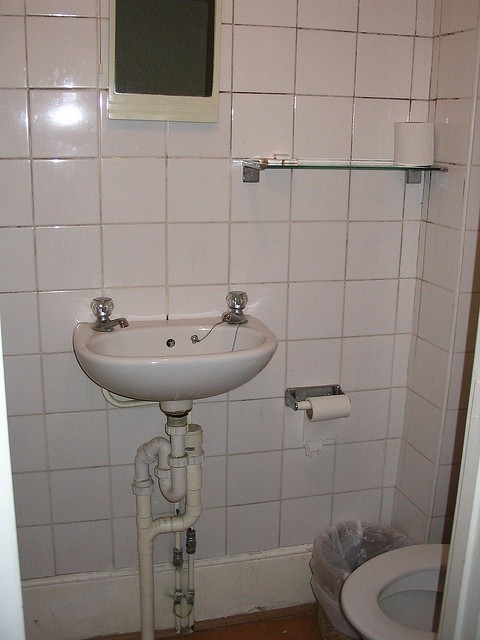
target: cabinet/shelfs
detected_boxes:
[242,157,447,185]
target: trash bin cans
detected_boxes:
[310,520,415,639]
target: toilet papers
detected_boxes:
[302,395,350,456]
[394,121,436,166]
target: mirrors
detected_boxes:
[107,0,221,124]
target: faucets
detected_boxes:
[90,298,130,332]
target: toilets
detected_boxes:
[340,543,451,639]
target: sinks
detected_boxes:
[73,315,278,361]
